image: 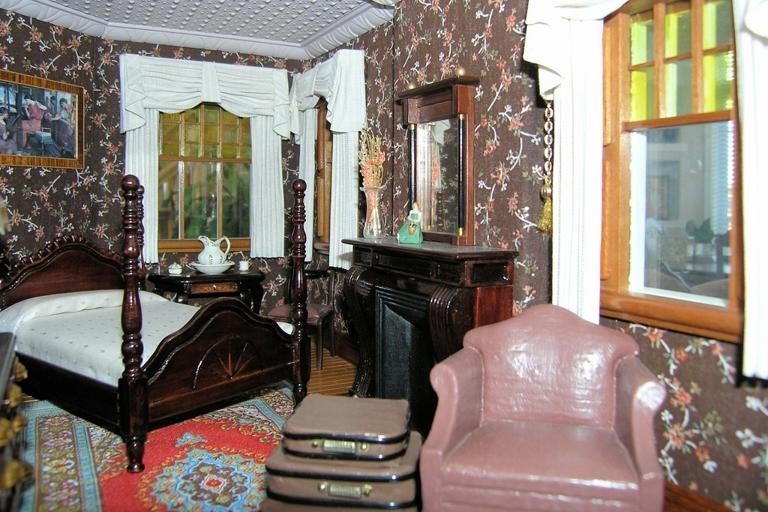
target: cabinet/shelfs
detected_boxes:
[338,235,521,433]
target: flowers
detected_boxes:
[354,128,391,233]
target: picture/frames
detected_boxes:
[0,68,87,172]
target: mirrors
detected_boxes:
[399,75,480,245]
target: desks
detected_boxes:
[145,269,266,316]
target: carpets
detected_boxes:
[9,383,296,510]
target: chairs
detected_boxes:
[416,295,671,512]
[268,267,336,371]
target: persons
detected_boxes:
[0,94,76,155]
[397,201,424,245]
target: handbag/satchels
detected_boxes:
[260,394,424,512]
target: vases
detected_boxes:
[356,182,388,239]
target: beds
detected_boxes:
[0,174,314,473]
